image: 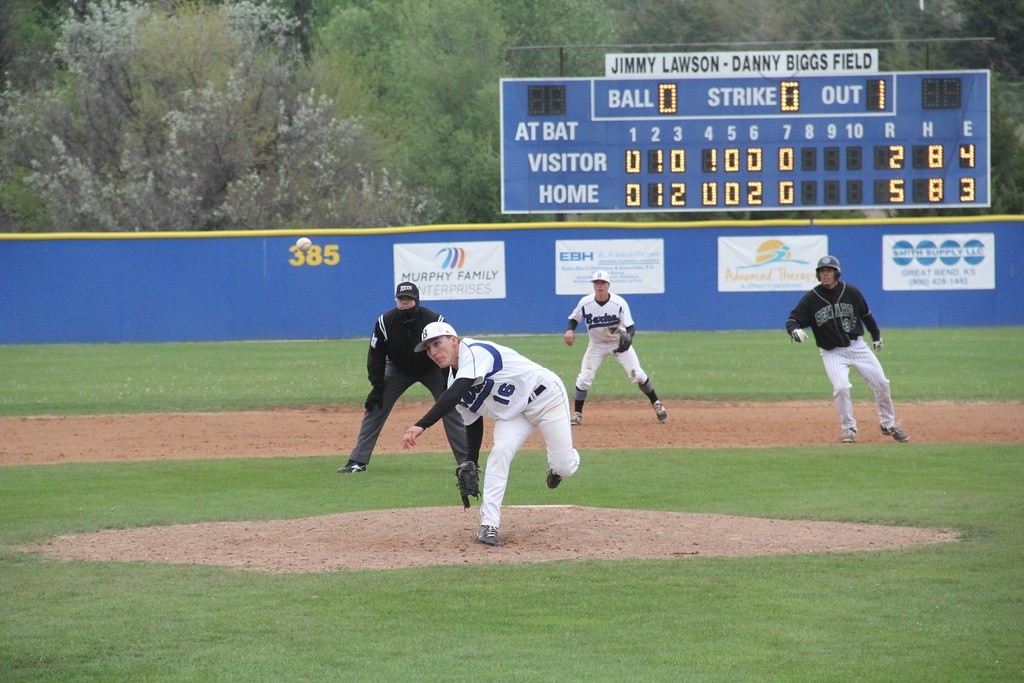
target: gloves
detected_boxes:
[791,328,807,343]
[365,387,383,412]
[873,338,884,350]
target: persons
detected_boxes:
[337,282,483,474]
[401,321,580,546]
[785,256,911,442]
[566,271,668,424]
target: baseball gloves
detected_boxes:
[454,461,478,508]
[613,329,632,353]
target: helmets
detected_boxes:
[816,256,841,282]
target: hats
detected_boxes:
[396,282,419,298]
[591,271,611,283]
[415,322,457,352]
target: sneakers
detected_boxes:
[839,428,858,442]
[547,469,561,489]
[570,412,583,425]
[338,460,367,472]
[653,399,668,421]
[881,425,910,442]
[476,525,498,545]
